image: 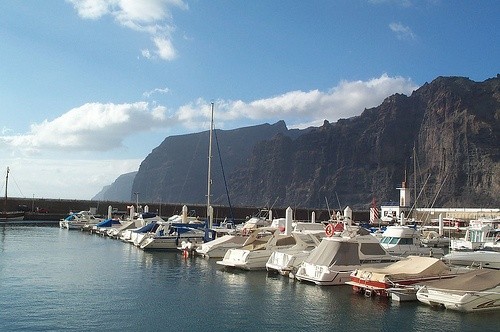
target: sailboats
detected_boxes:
[1,167,25,222]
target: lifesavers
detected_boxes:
[325,223,334,237]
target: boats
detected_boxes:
[416,268,500,313]
[58,100,499,275]
[293,220,403,285]
[345,257,478,302]
